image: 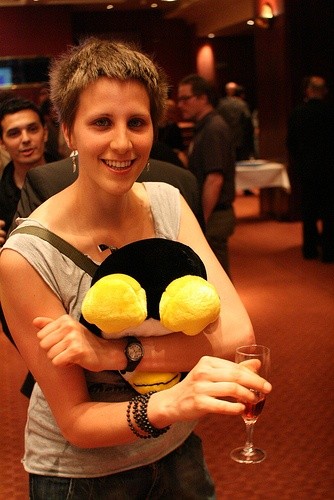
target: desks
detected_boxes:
[235,163,291,196]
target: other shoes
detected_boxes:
[305,250,319,258]
[323,251,334,261]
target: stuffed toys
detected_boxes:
[81,238,221,395]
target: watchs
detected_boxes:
[119,337,143,376]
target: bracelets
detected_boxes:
[127,391,169,439]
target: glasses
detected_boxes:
[176,93,196,103]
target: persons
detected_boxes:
[0,36,273,500]
[0,74,334,346]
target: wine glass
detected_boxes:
[230,345,271,464]
